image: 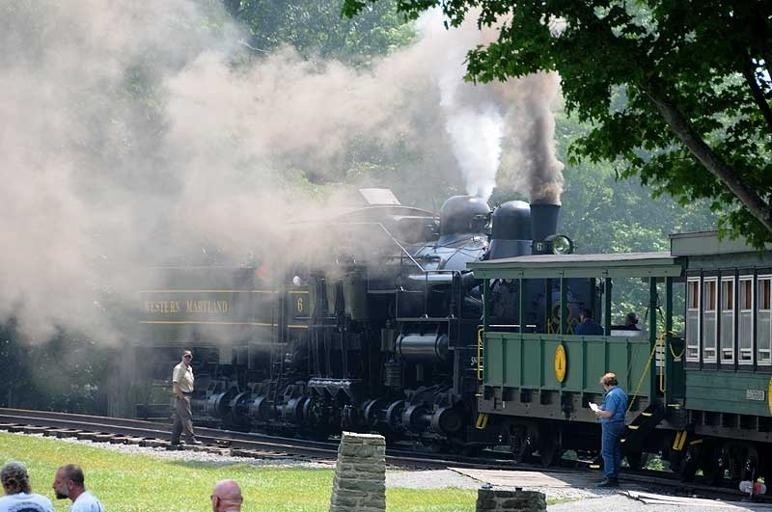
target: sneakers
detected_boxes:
[172,441,184,445]
[186,439,202,444]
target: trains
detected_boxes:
[100,181,768,495]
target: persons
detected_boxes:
[596,372,629,487]
[0,461,57,512]
[623,312,641,331]
[210,480,242,512]
[575,308,603,335]
[51,463,105,512]
[171,350,203,445]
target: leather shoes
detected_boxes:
[597,476,620,487]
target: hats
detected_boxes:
[182,350,192,358]
